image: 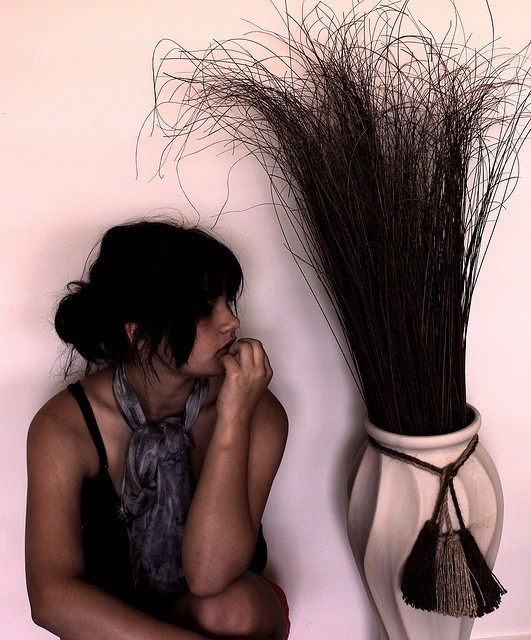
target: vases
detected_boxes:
[348,404,505,640]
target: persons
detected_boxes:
[25,222,290,640]
